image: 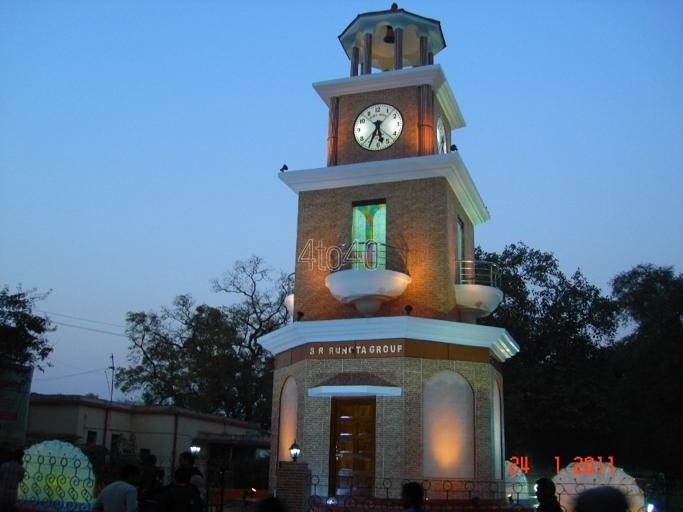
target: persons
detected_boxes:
[0,431,633,508]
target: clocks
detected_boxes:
[352,103,404,151]
